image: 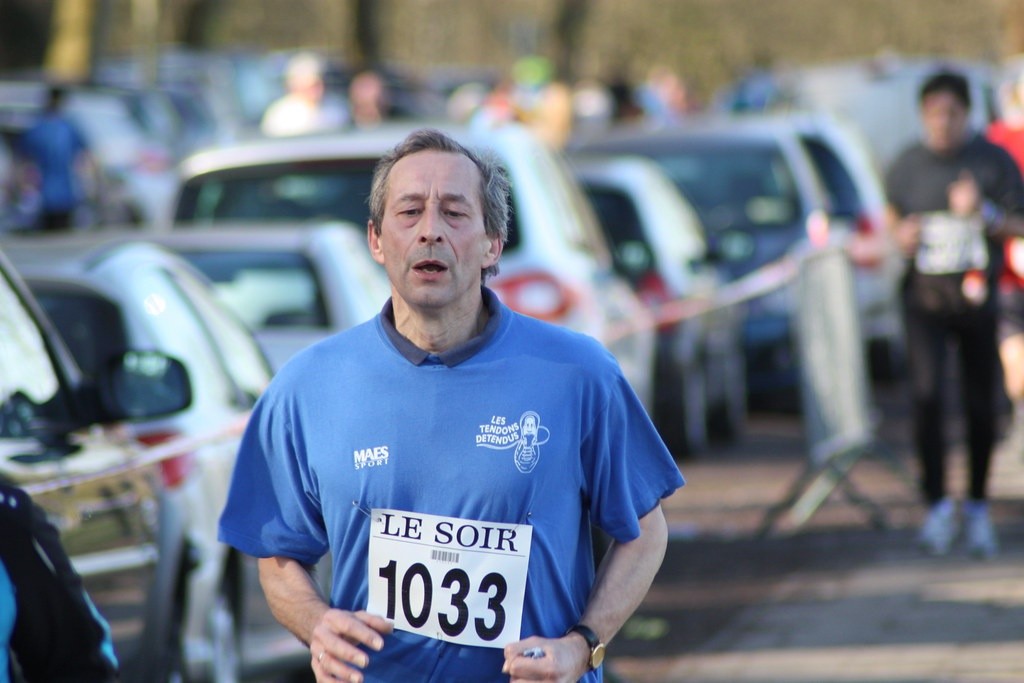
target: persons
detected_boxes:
[257,50,688,140]
[18,88,90,232]
[215,129,686,683]
[882,52,1024,555]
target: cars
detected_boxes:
[0,54,659,683]
[558,60,1018,455]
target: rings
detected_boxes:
[317,652,324,661]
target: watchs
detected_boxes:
[565,624,605,670]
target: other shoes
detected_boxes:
[921,499,955,555]
[965,498,995,558]
